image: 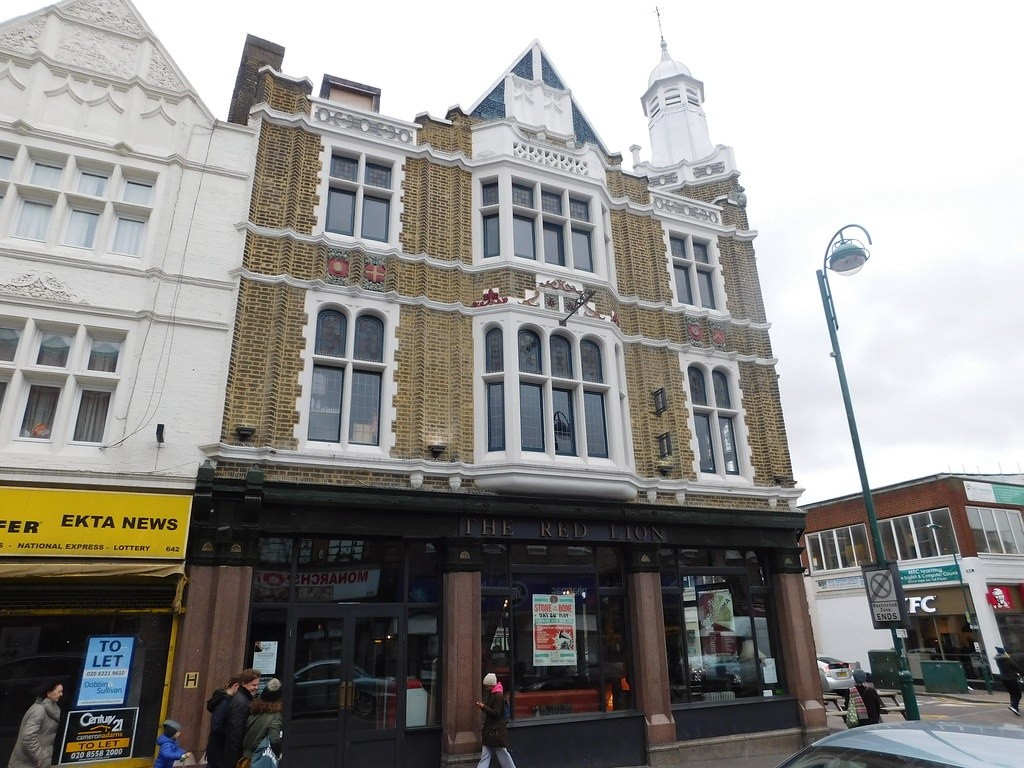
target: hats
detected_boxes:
[162,719,181,738]
[260,678,282,701]
[482,673,498,686]
[994,647,1005,654]
[853,670,866,683]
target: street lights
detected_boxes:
[816,220,927,721]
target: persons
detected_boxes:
[994,646,1022,716]
[223,670,260,768]
[8,680,63,768]
[845,669,881,726]
[204,674,243,768]
[152,719,191,768]
[475,673,517,768]
[236,678,283,768]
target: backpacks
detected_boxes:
[503,697,510,724]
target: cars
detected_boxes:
[481,663,612,722]
[688,654,750,688]
[281,657,374,720]
[770,721,1023,767]
[0,648,99,735]
[756,657,853,700]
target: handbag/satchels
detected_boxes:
[236,757,252,768]
[846,694,858,729]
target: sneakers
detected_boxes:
[1007,705,1022,716]
